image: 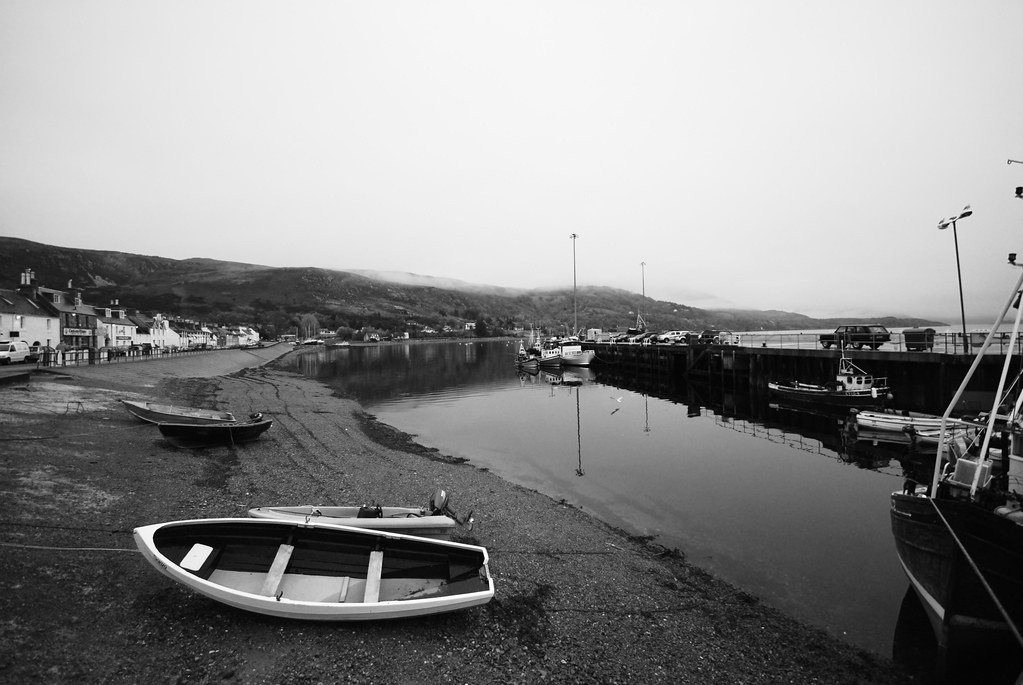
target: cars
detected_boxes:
[28,345,58,363]
[611,332,661,345]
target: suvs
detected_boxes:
[656,330,691,344]
[819,325,891,349]
[697,329,732,345]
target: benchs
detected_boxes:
[179,543,214,572]
[212,414,221,419]
[260,544,294,597]
[363,551,383,603]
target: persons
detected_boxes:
[56,339,69,366]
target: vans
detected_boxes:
[0,340,31,365]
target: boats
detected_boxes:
[120,398,238,427]
[157,411,274,449]
[513,322,598,369]
[766,338,894,403]
[836,159,1023,685]
[245,489,477,539]
[132,515,495,625]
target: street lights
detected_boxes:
[640,261,647,323]
[569,233,578,337]
[937,204,973,354]
